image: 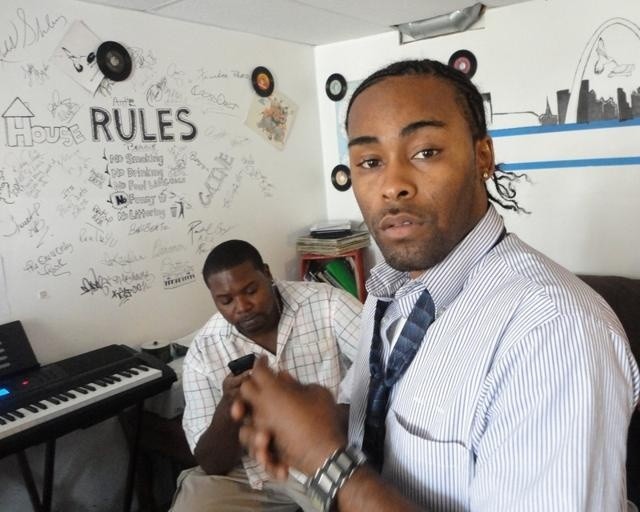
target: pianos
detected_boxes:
[1,343,178,458]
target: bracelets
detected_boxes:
[306,440,368,512]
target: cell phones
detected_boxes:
[226,354,257,374]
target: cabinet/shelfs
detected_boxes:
[299,249,365,304]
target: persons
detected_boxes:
[228,57,639,512]
[169,239,365,512]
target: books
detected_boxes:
[295,220,370,300]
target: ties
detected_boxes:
[361,289,435,464]
